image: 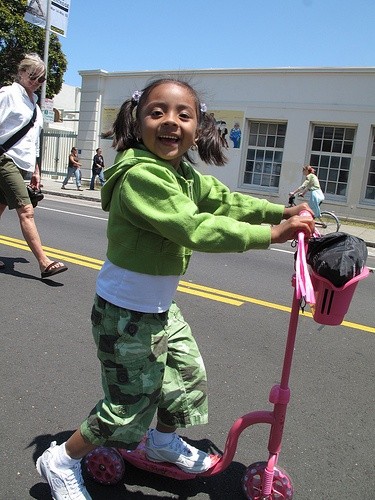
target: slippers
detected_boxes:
[41,260,69,279]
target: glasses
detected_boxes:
[25,70,46,84]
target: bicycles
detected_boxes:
[285,194,340,237]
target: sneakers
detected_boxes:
[35,441,91,500]
[146,428,222,474]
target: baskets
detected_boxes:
[314,268,369,326]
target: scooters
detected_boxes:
[85,210,370,500]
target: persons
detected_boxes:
[61,147,83,191]
[34,79,316,500]
[0,52,68,279]
[90,148,105,190]
[288,164,325,218]
